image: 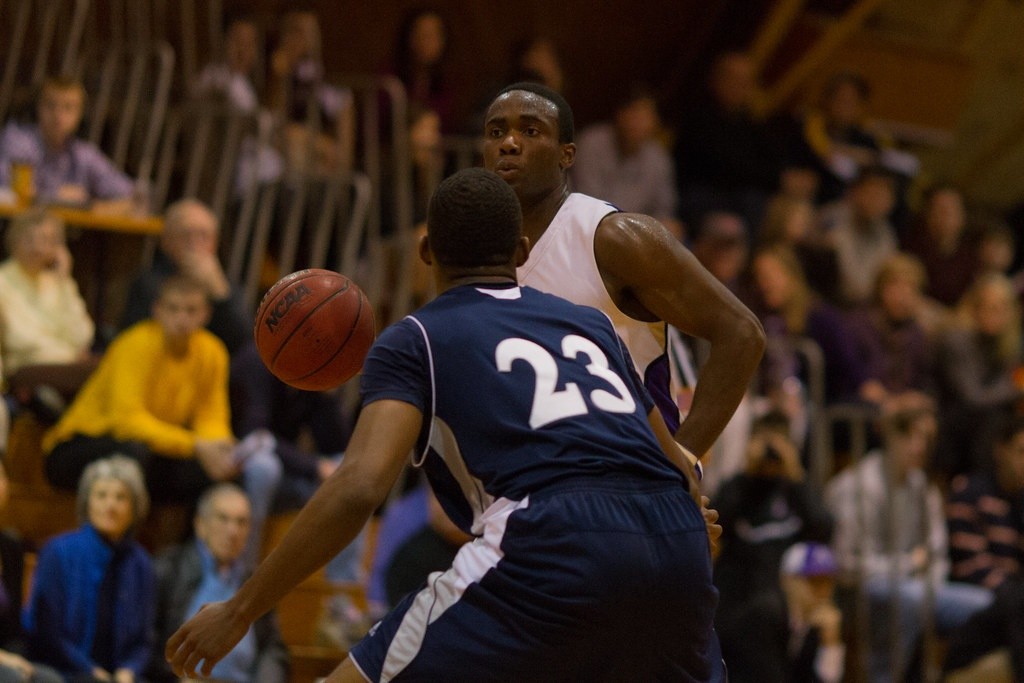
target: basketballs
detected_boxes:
[253,269,377,390]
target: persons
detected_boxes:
[166,171,727,683]
[485,85,765,482]
[1,11,1024,683]
